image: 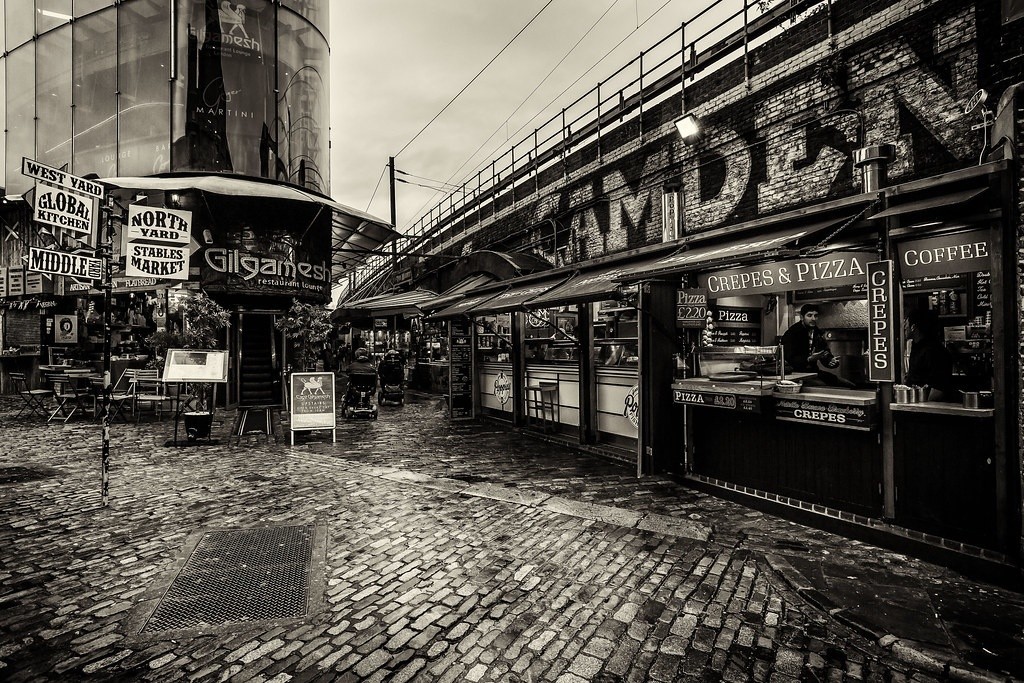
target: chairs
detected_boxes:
[9,364,169,423]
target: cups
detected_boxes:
[963,392,978,409]
[744,344,778,354]
[894,388,929,403]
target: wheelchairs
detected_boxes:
[377,351,406,406]
[341,371,379,420]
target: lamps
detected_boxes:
[674,113,707,143]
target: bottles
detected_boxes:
[497,353,510,362]
[478,336,494,347]
[36,346,41,354]
[9,347,13,357]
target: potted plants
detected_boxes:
[276,299,336,436]
[168,295,228,439]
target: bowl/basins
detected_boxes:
[776,382,803,393]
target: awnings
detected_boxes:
[330,182,991,323]
[92,176,405,291]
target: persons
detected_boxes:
[379,350,405,391]
[321,342,378,405]
[904,310,952,391]
[780,303,856,389]
